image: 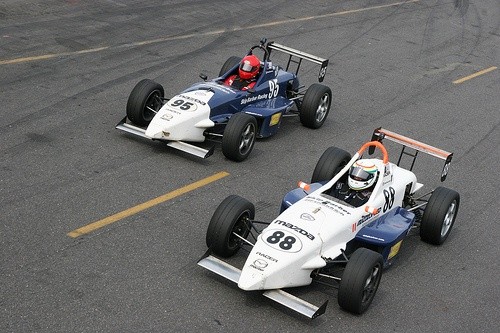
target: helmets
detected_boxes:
[238,55,260,80]
[348,158,378,191]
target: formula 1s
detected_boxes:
[196,126,461,321]
[114,37,333,162]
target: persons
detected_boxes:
[329,159,378,207]
[224,56,261,91]
